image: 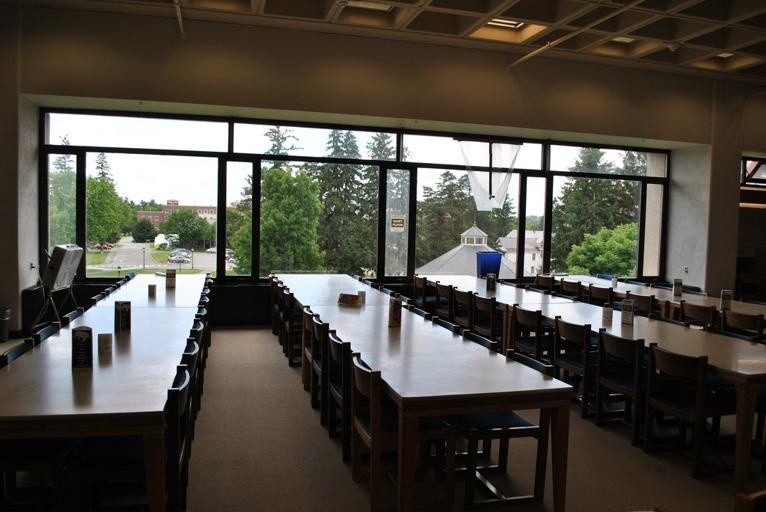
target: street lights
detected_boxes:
[190,247,195,269]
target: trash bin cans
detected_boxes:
[0,304,12,342]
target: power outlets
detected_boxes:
[29,262,34,271]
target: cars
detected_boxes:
[168,247,192,265]
[165,233,180,244]
[89,242,114,249]
[206,247,237,265]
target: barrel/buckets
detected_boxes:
[475,250,503,281]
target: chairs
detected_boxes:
[265,270,766,512]
[0,272,214,512]
